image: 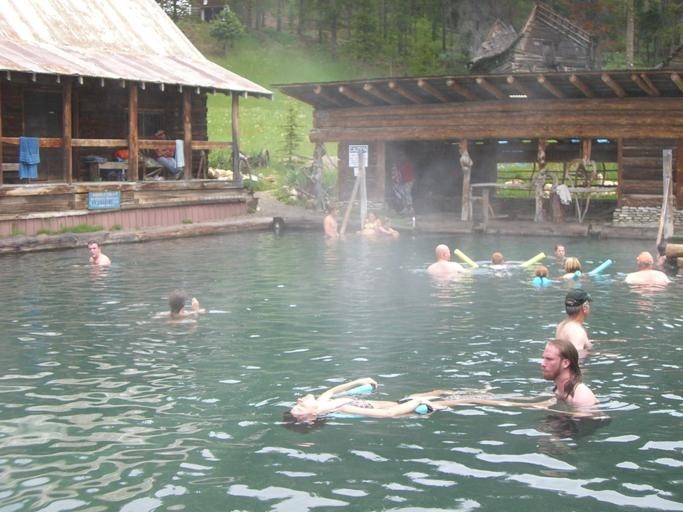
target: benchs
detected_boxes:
[86,159,168,180]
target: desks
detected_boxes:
[469,183,617,225]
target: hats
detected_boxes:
[564,288,592,307]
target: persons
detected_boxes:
[537,340,598,416]
[362,210,399,238]
[282,375,557,434]
[554,288,594,361]
[88,240,110,267]
[427,242,678,291]
[393,147,418,215]
[154,289,200,315]
[323,207,340,241]
[153,129,184,179]
